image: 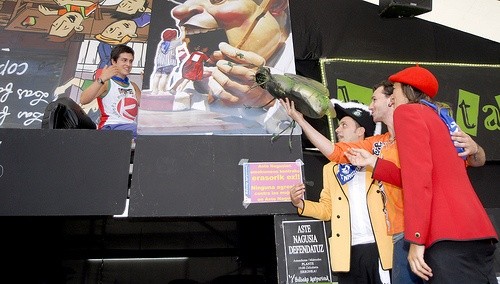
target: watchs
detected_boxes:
[97,77,104,85]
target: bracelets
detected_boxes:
[469,143,480,161]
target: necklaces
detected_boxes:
[389,136,395,142]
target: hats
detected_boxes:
[334,103,376,137]
[388,64,439,99]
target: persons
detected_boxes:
[279,64,500,284]
[80,44,141,144]
